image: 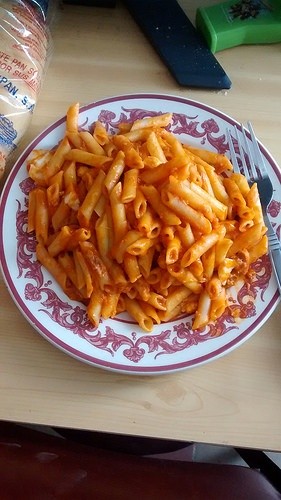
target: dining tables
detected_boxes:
[0,0,281,453]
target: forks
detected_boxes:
[226,121,280,295]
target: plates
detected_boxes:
[1,95,280,376]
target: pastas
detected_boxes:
[28,103,269,343]
